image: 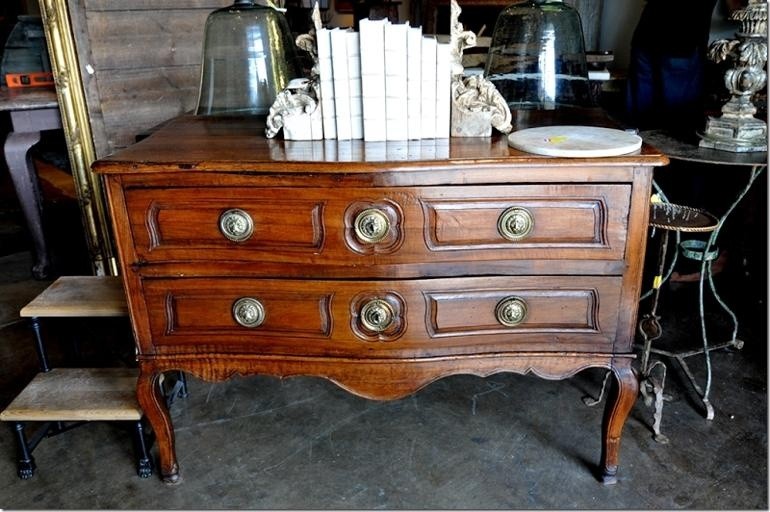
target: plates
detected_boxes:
[506,124,645,158]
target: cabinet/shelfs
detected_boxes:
[90,108,669,484]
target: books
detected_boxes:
[317,18,452,141]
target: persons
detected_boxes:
[624,0,744,128]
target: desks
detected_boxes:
[1,88,59,281]
[583,126,769,448]
